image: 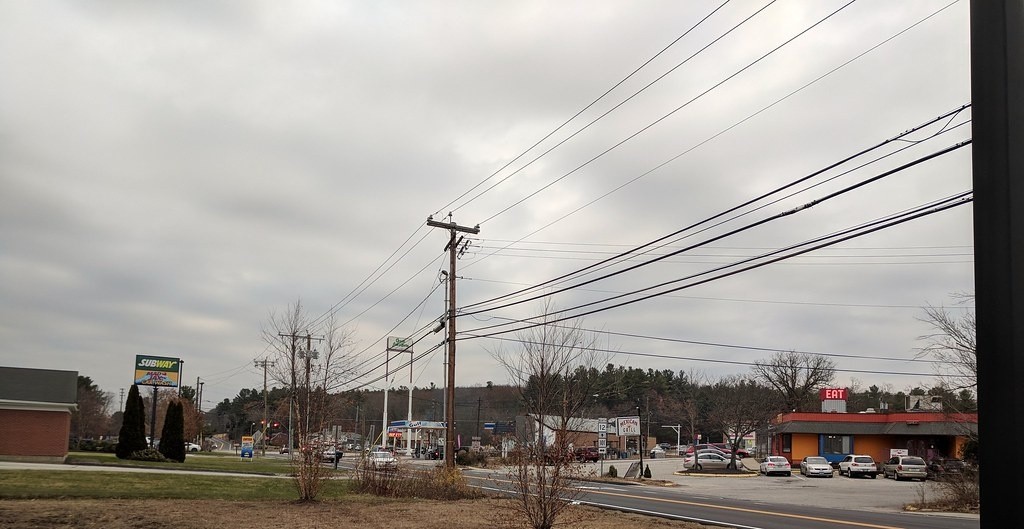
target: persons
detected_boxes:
[567,441,574,463]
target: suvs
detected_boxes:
[928,457,964,481]
[838,453,876,479]
[883,455,929,482]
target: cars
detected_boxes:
[320,448,343,463]
[279,445,289,455]
[649,441,749,473]
[572,446,600,463]
[758,455,792,477]
[800,456,834,478]
[366,452,399,471]
[184,442,202,453]
[430,446,445,460]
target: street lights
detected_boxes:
[442,269,448,460]
[250,423,256,438]
[178,360,186,399]
[199,381,205,412]
